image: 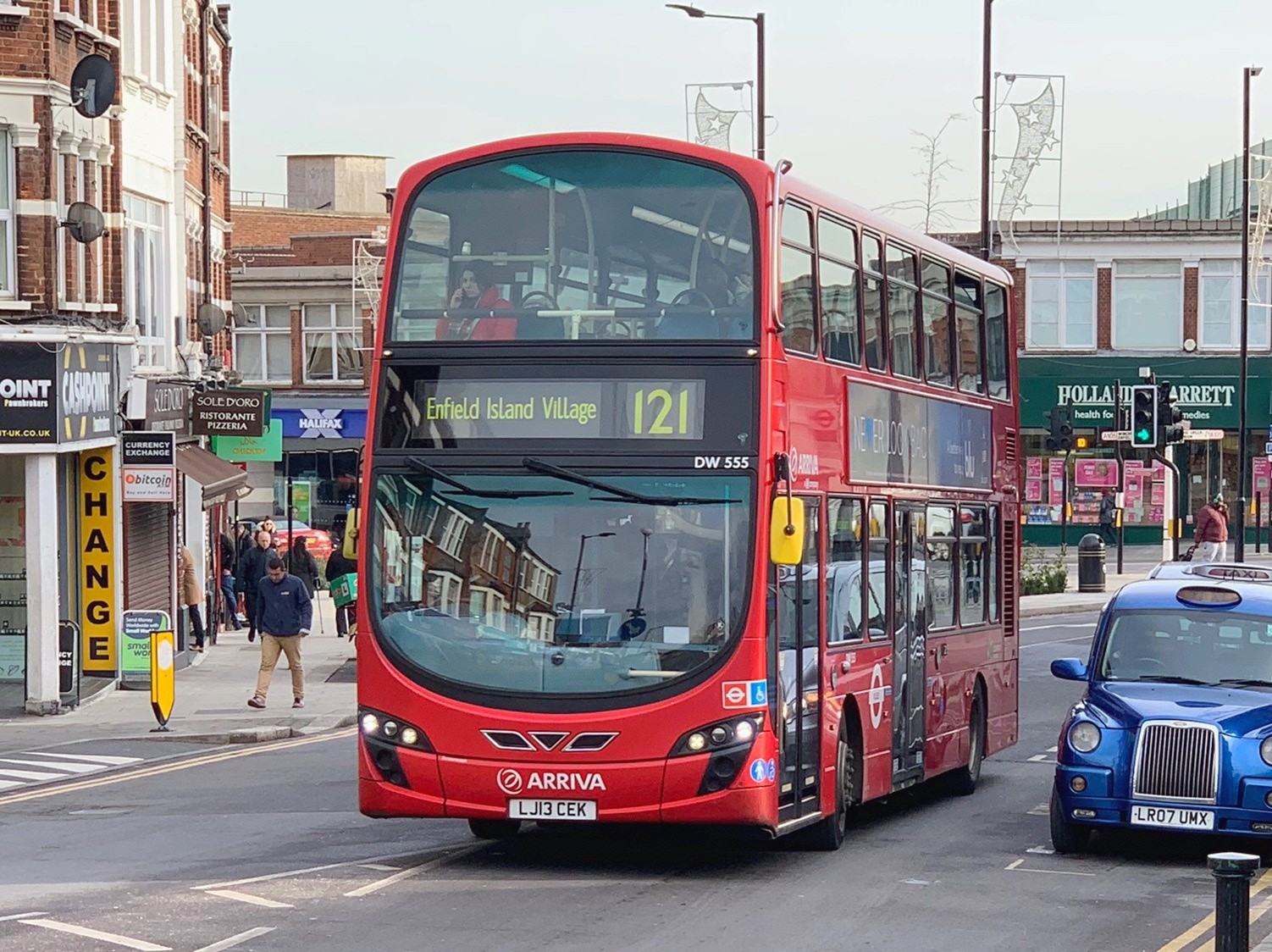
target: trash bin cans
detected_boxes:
[1078,534,1106,592]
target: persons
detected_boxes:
[236,531,282,642]
[247,557,313,709]
[436,258,517,340]
[1194,494,1230,561]
[177,536,204,653]
[697,249,753,337]
[215,516,281,630]
[290,506,299,521]
[596,526,682,611]
[280,536,321,598]
[1097,487,1119,547]
[324,534,357,637]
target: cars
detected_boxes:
[233,515,334,571]
[1145,560,1272,587]
[1046,577,1272,864]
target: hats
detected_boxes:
[1101,487,1109,495]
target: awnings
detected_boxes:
[175,444,254,511]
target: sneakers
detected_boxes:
[248,697,265,708]
[292,698,304,707]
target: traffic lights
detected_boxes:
[1131,385,1157,447]
[1163,402,1185,445]
[1076,436,1087,449]
[1044,407,1074,450]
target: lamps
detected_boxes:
[156,377,228,393]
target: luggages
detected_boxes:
[1177,545,1195,562]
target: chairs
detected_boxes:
[515,288,856,364]
[1135,615,1189,671]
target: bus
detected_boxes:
[344,132,1025,854]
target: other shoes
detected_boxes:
[338,631,344,638]
[189,644,203,653]
[248,627,257,642]
[232,619,243,630]
[1111,544,1118,548]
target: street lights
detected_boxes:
[665,2,767,161]
